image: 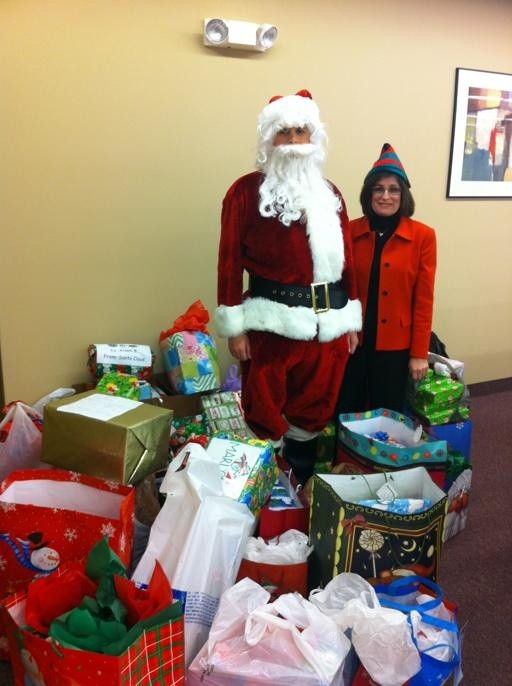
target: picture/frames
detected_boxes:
[445,65,512,198]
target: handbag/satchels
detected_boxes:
[0,330,471,686]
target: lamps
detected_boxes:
[203,16,280,52]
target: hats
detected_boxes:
[367,144,410,188]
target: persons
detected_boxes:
[215,90,364,487]
[350,142,437,414]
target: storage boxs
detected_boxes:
[40,389,174,487]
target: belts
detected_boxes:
[249,274,348,313]
